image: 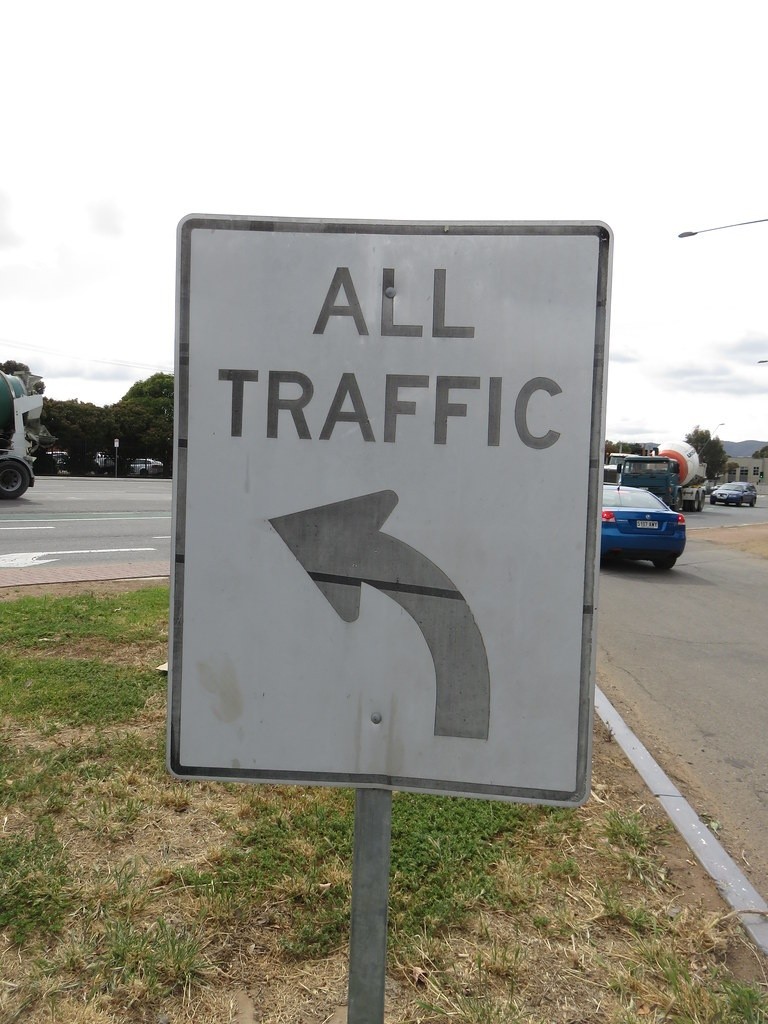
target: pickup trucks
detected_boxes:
[94,458,114,475]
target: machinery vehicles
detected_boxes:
[0,368,45,503]
[616,441,708,512]
[604,453,643,486]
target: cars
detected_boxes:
[130,458,164,476]
[600,484,686,568]
[710,485,721,490]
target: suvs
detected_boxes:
[710,482,757,507]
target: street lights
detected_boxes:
[698,423,725,455]
